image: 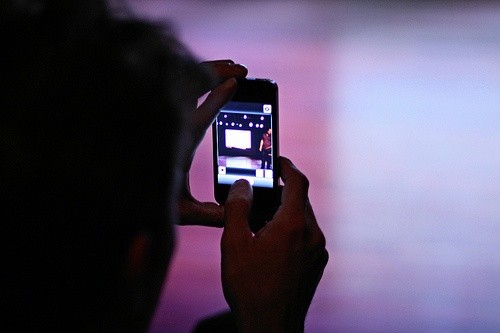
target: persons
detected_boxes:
[0,0,329,332]
[258,127,272,168]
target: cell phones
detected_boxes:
[212,78,280,211]
